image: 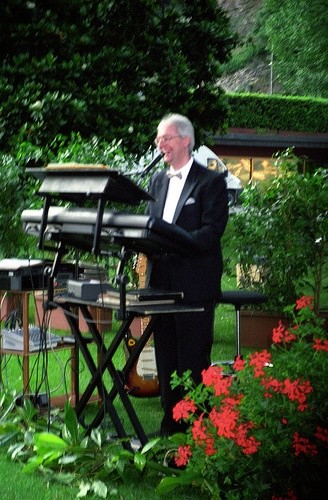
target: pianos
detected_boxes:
[20,206,202,256]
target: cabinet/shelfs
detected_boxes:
[0,289,104,416]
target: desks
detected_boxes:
[50,295,204,464]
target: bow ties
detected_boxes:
[166,171,182,179]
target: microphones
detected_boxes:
[140,152,164,178]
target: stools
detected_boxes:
[212,291,275,378]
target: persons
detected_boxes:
[145,112,229,442]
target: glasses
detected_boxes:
[155,136,182,146]
